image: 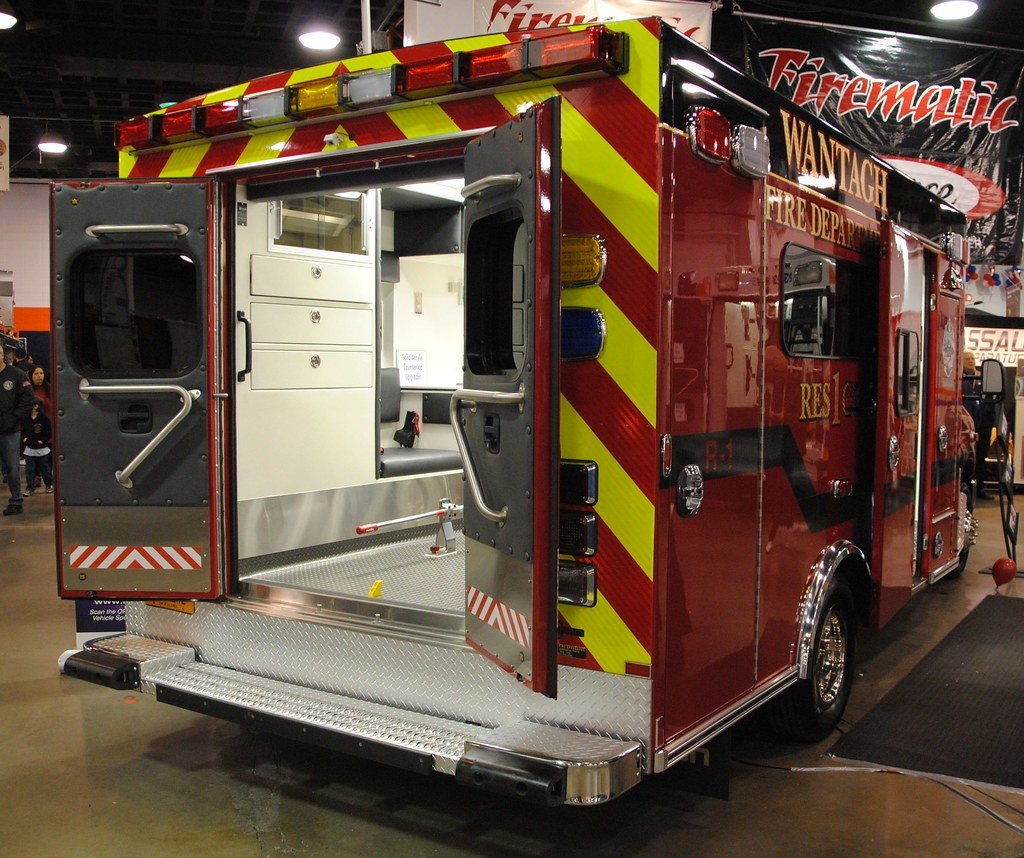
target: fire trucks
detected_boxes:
[48,15,1007,806]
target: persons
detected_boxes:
[962,353,995,499]
[0,347,34,517]
[23,402,53,496]
[5,345,51,484]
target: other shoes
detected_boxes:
[976,491,994,500]
[3,505,23,515]
[3,476,7,482]
[23,489,31,497]
[33,484,42,488]
[46,488,54,493]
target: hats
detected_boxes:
[14,347,26,358]
